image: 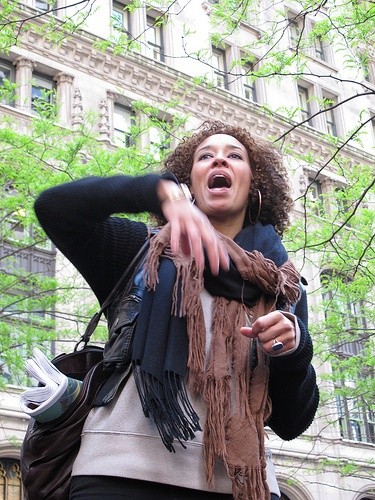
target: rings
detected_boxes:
[272,338,284,351]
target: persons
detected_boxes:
[33,119,320,500]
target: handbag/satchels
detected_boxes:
[19,234,156,500]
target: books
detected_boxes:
[19,346,84,423]
